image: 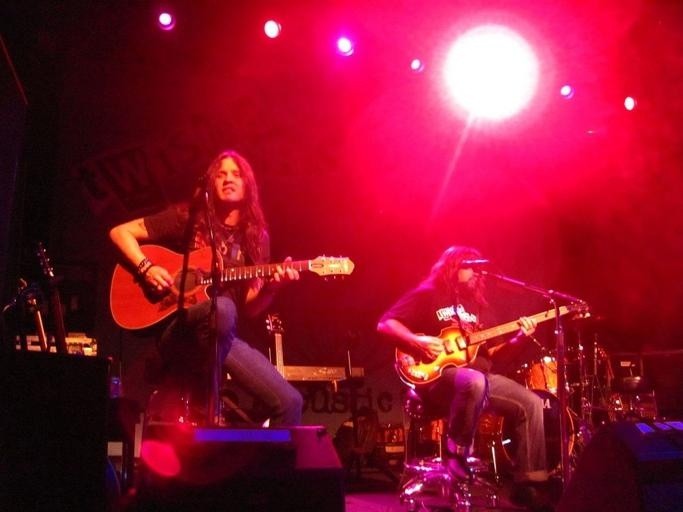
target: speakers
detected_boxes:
[553,418,683,512]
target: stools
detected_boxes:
[397,381,505,512]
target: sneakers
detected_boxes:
[512,484,553,511]
[441,449,470,482]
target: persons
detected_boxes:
[108,153,303,512]
[377,245,557,512]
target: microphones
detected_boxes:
[197,176,208,202]
[461,258,490,268]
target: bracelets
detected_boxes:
[137,257,155,279]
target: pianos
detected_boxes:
[15,335,98,358]
[273,365,365,382]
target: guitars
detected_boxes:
[109,244,355,330]
[0,241,85,354]
[393,299,590,390]
[265,315,284,379]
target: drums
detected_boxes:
[492,390,576,479]
[525,358,558,396]
[612,354,644,386]
